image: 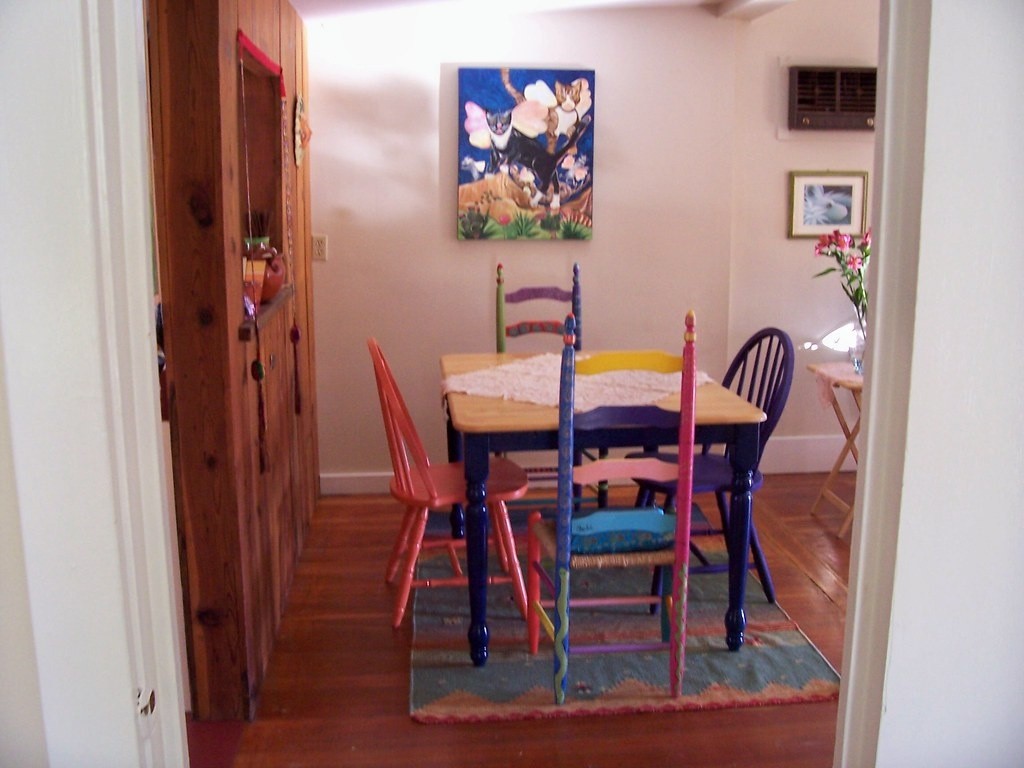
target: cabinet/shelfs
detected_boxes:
[148,0,319,721]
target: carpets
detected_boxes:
[409,502,841,723]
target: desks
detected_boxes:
[806,362,863,538]
[441,351,767,668]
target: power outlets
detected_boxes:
[312,235,328,259]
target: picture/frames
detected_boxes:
[789,171,868,238]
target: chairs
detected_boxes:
[368,262,794,627]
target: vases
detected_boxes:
[848,321,868,372]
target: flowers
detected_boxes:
[813,227,872,339]
